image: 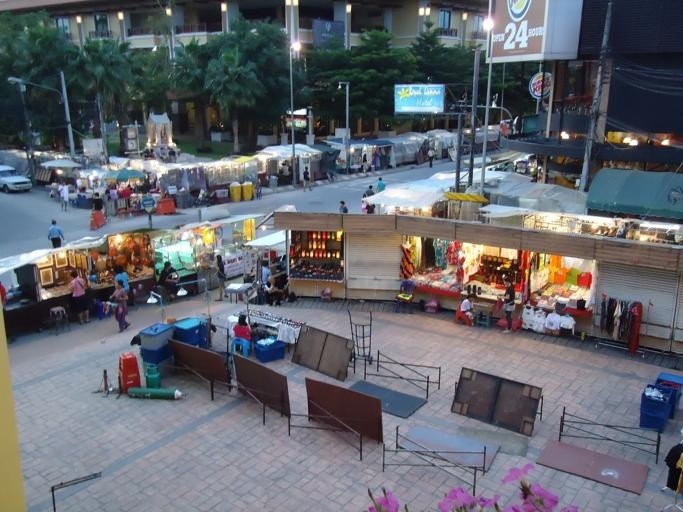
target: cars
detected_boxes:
[1,166,31,195]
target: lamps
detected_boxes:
[176,279,211,318]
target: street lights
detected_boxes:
[338,81,350,140]
[7,72,77,157]
[289,42,301,186]
[19,85,34,151]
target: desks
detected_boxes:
[226,283,244,304]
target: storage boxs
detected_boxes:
[655,372,683,420]
[138,323,174,350]
[254,341,286,363]
[174,318,209,347]
[474,303,493,314]
[140,345,173,363]
[639,384,674,433]
[142,354,177,379]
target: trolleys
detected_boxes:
[345,308,376,367]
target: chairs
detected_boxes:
[321,287,332,301]
[147,290,164,324]
[132,284,148,312]
[455,304,472,326]
[231,337,251,357]
[155,285,171,303]
[393,279,416,313]
[425,298,440,314]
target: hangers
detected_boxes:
[661,430,683,512]
[594,288,651,359]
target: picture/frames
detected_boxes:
[81,255,87,270]
[55,252,68,268]
[76,253,82,267]
[40,267,54,287]
[87,256,92,270]
[68,251,76,269]
[37,255,53,268]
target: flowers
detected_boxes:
[368,463,577,512]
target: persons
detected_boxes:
[460,294,478,326]
[68,271,91,324]
[281,163,290,184]
[263,276,282,306]
[109,281,130,332]
[428,148,433,167]
[159,261,180,299]
[499,278,515,332]
[48,219,64,249]
[363,177,386,216]
[662,444,683,493]
[58,183,69,208]
[304,166,310,187]
[528,155,537,180]
[94,182,120,219]
[216,255,229,301]
[231,315,252,358]
[340,200,344,211]
[114,267,133,309]
[362,153,368,174]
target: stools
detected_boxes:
[476,311,493,327]
[96,299,110,320]
[50,306,70,335]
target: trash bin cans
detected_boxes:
[231,180,241,203]
[243,219,255,240]
[242,183,255,203]
[639,384,678,431]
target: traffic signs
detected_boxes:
[485,0,583,63]
[142,192,156,228]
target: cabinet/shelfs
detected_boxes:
[286,231,345,299]
[117,194,161,218]
[406,235,463,311]
[461,243,522,319]
[671,328,683,354]
[156,270,198,302]
[40,285,76,330]
[169,186,201,208]
[77,190,94,208]
[199,251,243,290]
[227,309,302,352]
[518,249,595,337]
[596,315,672,353]
[260,266,288,300]
[3,299,42,344]
[50,175,75,185]
[209,183,232,204]
[91,275,153,318]
[258,170,293,187]
[346,279,404,300]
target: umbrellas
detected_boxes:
[103,168,145,179]
